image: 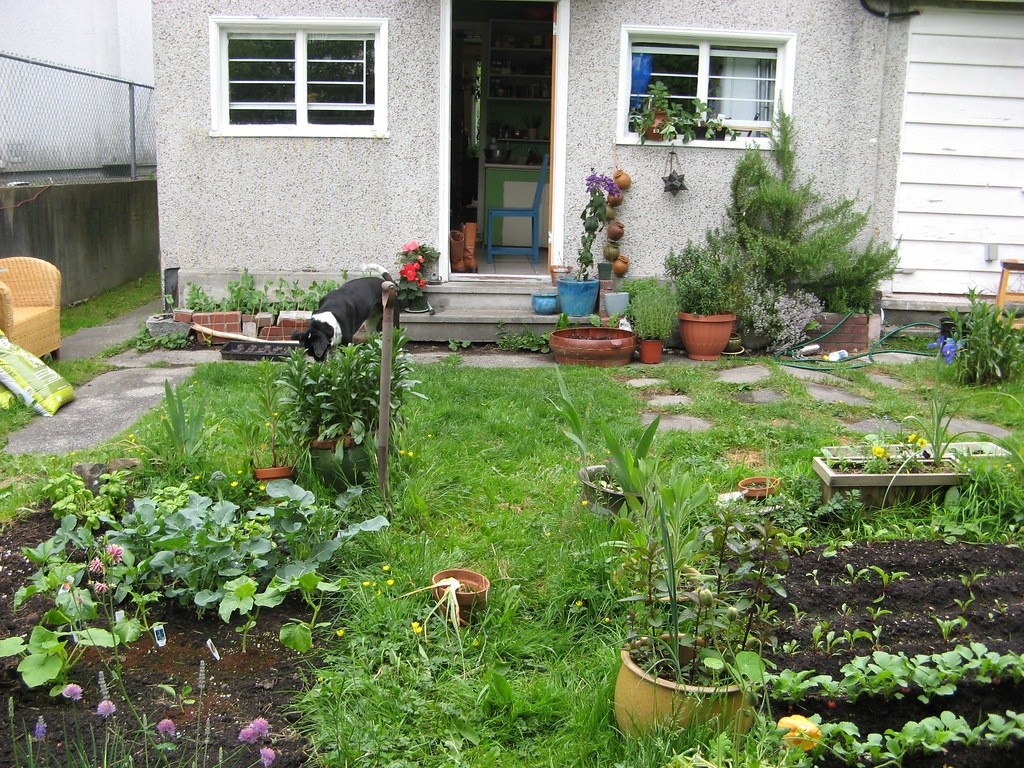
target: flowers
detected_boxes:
[750,288,828,355]
[929,335,964,365]
[860,425,928,475]
[577,167,620,281]
[395,242,440,300]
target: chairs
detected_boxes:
[0,256,71,361]
[487,154,549,263]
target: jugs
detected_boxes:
[510,128,524,138]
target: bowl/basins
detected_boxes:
[484,148,511,163]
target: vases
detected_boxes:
[531,292,559,315]
[605,292,630,317]
[556,277,600,317]
[549,326,637,368]
[661,239,753,360]
[407,295,428,311]
[738,477,780,503]
[725,332,742,353]
[597,262,612,279]
[739,323,774,350]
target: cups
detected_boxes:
[528,128,537,139]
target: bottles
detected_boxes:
[492,56,511,73]
[490,138,498,149]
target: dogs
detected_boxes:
[289,264,400,367]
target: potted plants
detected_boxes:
[630,80,682,145]
[273,325,429,493]
[219,340,298,360]
[388,568,490,682]
[705,121,741,141]
[632,285,680,363]
[577,464,655,520]
[613,510,791,744]
[812,379,1024,509]
[676,98,714,144]
[229,355,300,485]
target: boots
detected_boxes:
[460,222,477,273]
[450,230,465,272]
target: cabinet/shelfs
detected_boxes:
[477,18,553,248]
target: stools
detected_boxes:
[994,259,1024,330]
